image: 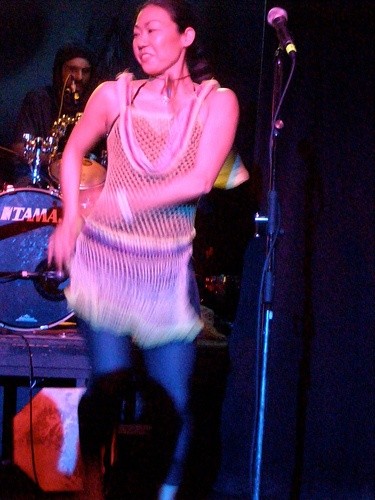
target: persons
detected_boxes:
[48,0,241,500]
[10,48,94,163]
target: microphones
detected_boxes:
[70,79,83,118]
[267,7,296,54]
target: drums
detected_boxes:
[0,186,88,332]
[47,115,109,190]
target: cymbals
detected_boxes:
[0,146,31,176]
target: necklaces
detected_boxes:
[153,74,191,81]
